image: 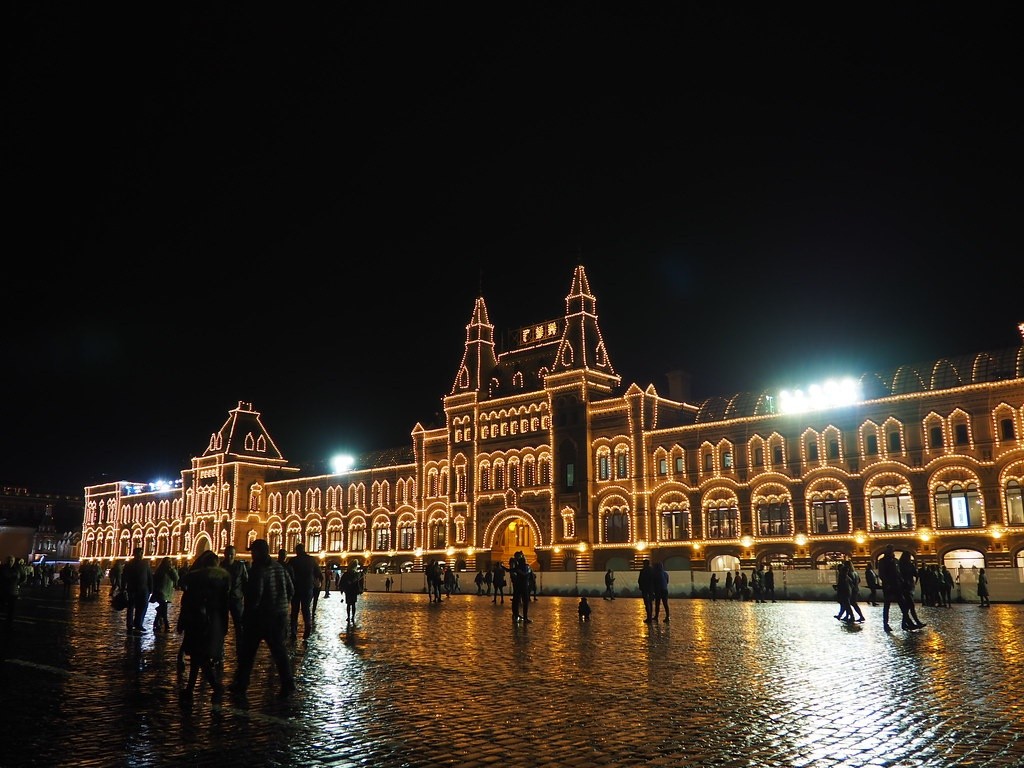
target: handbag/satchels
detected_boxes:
[111,592,127,611]
[352,572,364,594]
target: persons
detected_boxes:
[603,569,615,600]
[475,551,538,624]
[0,538,375,702]
[710,574,719,601]
[834,544,954,632]
[725,565,776,603]
[385,578,390,592]
[978,568,990,607]
[638,560,669,622]
[425,559,461,603]
[578,598,591,622]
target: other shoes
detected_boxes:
[857,618,865,621]
[834,616,840,620]
[223,684,247,694]
[903,626,909,630]
[909,624,918,630]
[885,627,893,631]
[276,688,296,699]
[917,623,927,629]
[179,689,193,697]
[841,619,847,621]
[212,689,225,698]
[134,623,145,630]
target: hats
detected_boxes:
[246,539,269,554]
